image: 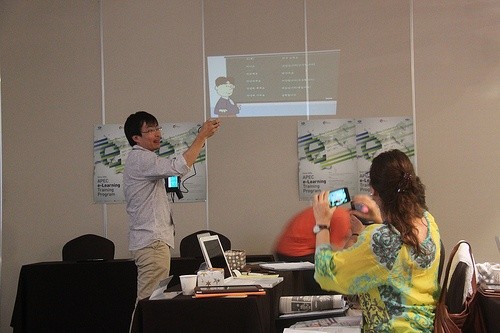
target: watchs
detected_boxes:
[313,224,331,234]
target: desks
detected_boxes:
[477,284,500,333]
[10,253,276,333]
[130,269,325,333]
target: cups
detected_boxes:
[179,275,198,296]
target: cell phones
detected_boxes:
[326,187,351,207]
[166,176,180,192]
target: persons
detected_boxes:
[116,111,223,332]
[312,149,441,333]
[273,204,356,293]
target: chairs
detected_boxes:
[433,240,487,333]
[180,230,232,258]
[61,233,115,262]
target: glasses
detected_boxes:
[141,127,162,135]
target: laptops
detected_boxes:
[200,235,284,289]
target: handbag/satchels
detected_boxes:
[434,240,488,333]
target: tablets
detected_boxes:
[195,283,264,293]
